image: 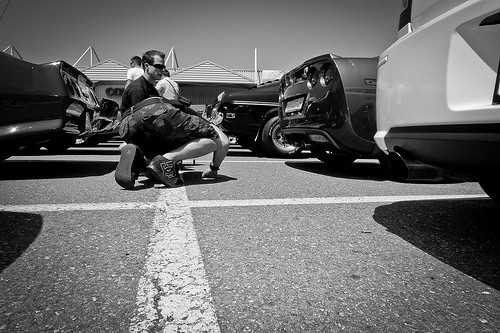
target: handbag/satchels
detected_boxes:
[178,95,191,107]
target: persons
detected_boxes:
[120,50,166,118]
[156,69,179,103]
[202,104,212,122]
[115,96,231,190]
[211,90,231,135]
[121,56,144,97]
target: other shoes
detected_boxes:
[147,154,185,187]
[114,144,145,190]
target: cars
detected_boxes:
[205,77,306,157]
[372,0,500,203]
[277,53,408,182]
[0,51,120,162]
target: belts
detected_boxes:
[121,98,168,123]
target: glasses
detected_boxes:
[152,64,166,69]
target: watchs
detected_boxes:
[209,163,220,171]
